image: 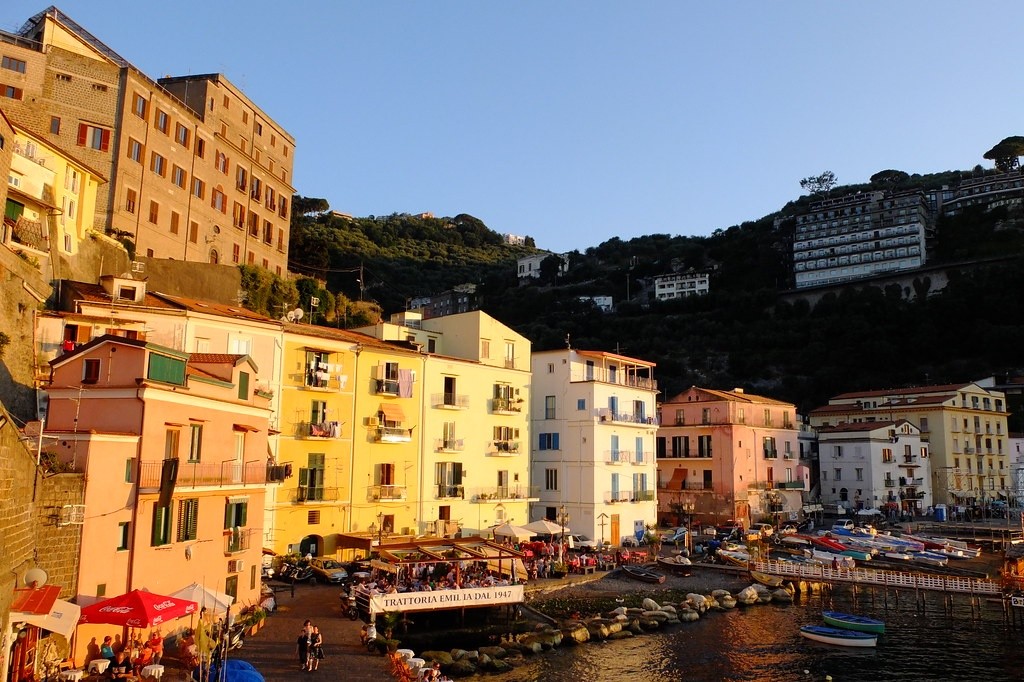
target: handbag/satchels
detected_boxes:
[318,647,325,659]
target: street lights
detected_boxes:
[557,504,570,542]
[773,492,781,533]
[369,512,387,545]
[682,498,695,556]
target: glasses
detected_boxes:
[153,635,156,636]
[133,636,136,638]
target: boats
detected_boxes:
[751,571,783,587]
[657,557,692,570]
[800,625,878,646]
[779,528,982,568]
[822,612,886,634]
[622,564,666,584]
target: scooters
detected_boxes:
[339,592,358,618]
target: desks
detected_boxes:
[141,664,166,682]
[602,554,613,561]
[116,647,150,660]
[89,659,111,682]
[60,670,84,682]
[632,552,648,555]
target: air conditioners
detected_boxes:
[229,559,245,572]
[367,416,379,426]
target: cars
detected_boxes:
[308,556,349,584]
[566,534,597,553]
[831,518,855,529]
[713,526,743,542]
[660,527,690,544]
[744,523,773,541]
[778,524,797,535]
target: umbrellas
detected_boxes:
[190,659,265,682]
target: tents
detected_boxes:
[76,588,198,650]
[523,518,570,544]
[172,582,235,632]
[482,523,538,544]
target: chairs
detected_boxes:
[528,548,655,578]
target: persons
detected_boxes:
[419,660,450,682]
[102,632,164,682]
[359,541,630,597]
[294,620,325,672]
[832,556,849,570]
[673,528,744,561]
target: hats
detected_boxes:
[104,636,112,642]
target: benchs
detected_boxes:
[579,566,596,574]
[602,562,616,569]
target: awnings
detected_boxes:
[9,584,81,644]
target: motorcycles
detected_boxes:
[278,560,313,582]
[261,563,275,579]
